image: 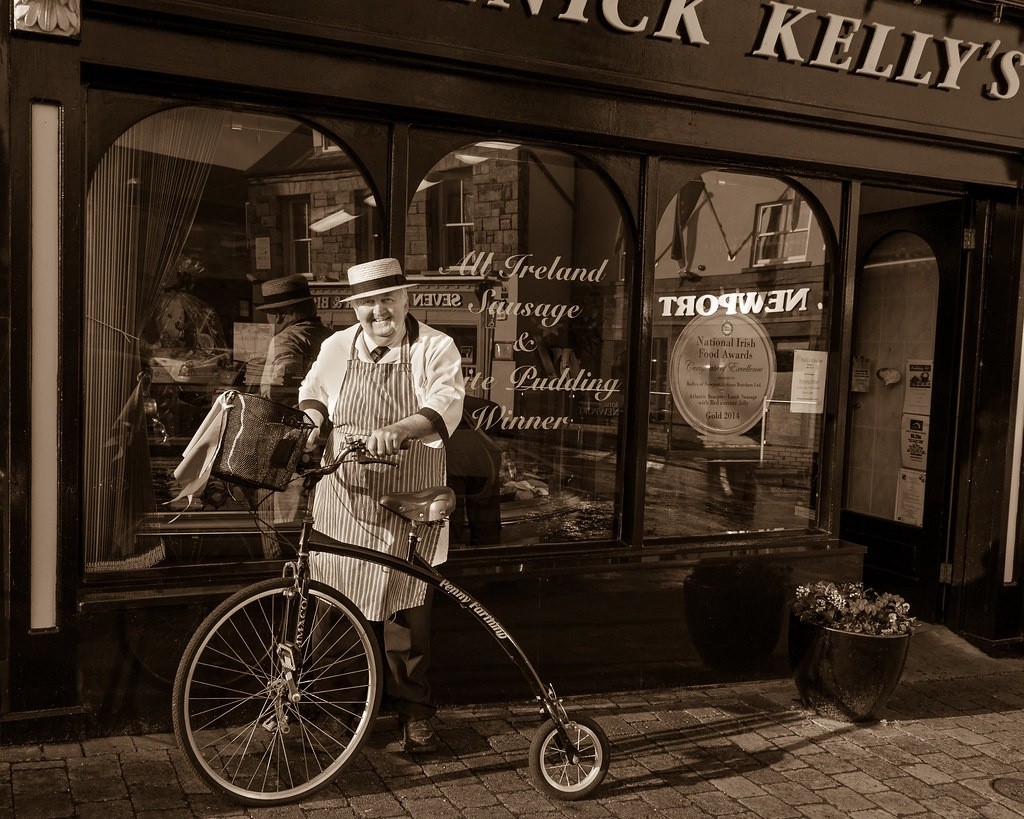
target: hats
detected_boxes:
[255,274,316,311]
[339,259,419,303]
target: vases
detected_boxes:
[154,291,192,346]
[786,610,914,726]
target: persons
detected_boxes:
[295,258,465,756]
[254,273,329,523]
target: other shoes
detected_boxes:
[299,713,353,751]
[403,717,438,754]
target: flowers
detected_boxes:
[162,255,203,293]
[790,576,920,639]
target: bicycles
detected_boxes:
[170,389,612,808]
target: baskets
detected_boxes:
[210,390,315,493]
